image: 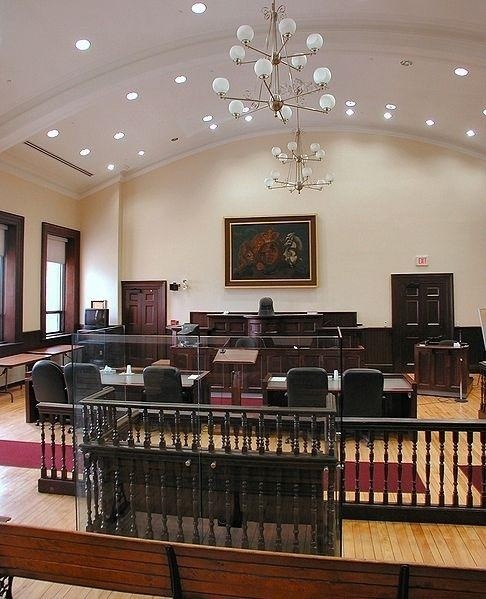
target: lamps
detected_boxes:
[209,1,335,191]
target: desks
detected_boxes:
[170,343,365,390]
[263,371,416,445]
[0,341,84,404]
[26,366,211,430]
[414,342,472,398]
[206,313,324,335]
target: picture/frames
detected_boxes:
[223,216,319,289]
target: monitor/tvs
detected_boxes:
[177,323,200,345]
[84,309,108,330]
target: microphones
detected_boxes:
[220,338,231,353]
[458,329,462,343]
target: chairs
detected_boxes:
[257,297,274,314]
[141,365,186,434]
[283,365,385,452]
[235,339,256,348]
[30,360,104,431]
